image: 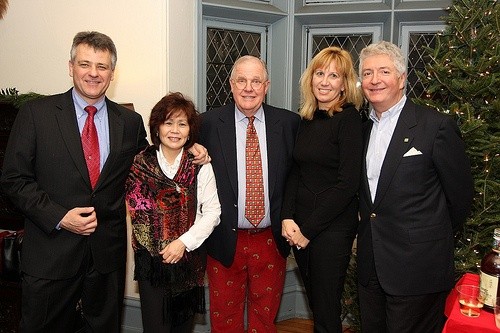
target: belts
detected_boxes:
[237,225,272,234]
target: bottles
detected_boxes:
[479,228,500,314]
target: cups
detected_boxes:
[493,297,500,328]
[457,286,488,317]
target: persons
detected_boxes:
[281,47,367,333]
[125,91,222,333]
[356,40,474,333]
[193,55,301,333]
[3,32,211,333]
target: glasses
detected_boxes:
[231,78,267,89]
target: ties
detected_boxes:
[245,115,265,227]
[81,106,101,191]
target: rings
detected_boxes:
[285,239,289,241]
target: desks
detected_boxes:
[441,273,500,333]
